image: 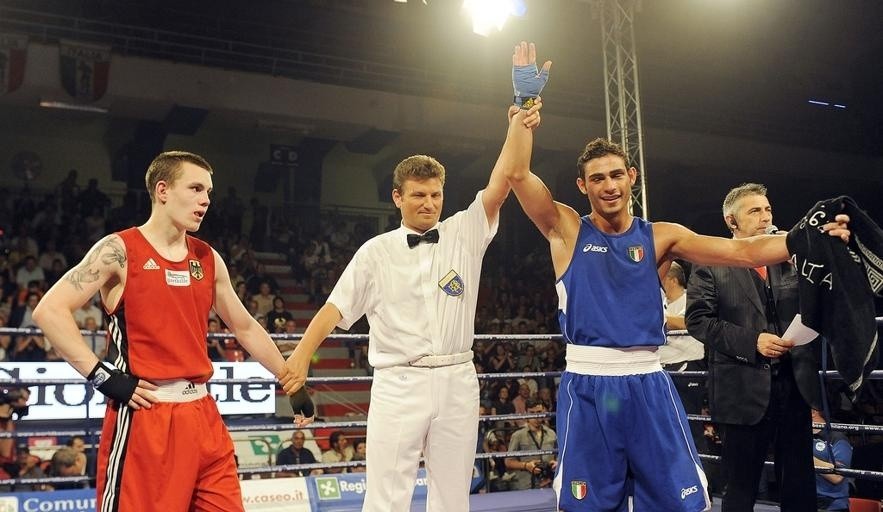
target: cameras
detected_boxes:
[535,463,553,479]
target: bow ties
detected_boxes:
[404,227,441,251]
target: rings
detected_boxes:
[773,350,775,356]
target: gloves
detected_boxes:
[85,359,144,412]
[287,385,317,418]
[511,60,551,103]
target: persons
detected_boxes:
[53,167,81,197]
[275,430,320,477]
[1,183,300,362]
[80,177,106,203]
[274,94,544,511]
[323,220,374,378]
[323,431,354,474]
[346,439,366,473]
[661,261,717,495]
[810,398,855,511]
[2,384,97,494]
[471,242,566,496]
[507,40,851,511]
[683,184,828,511]
[299,232,317,306]
[31,152,317,511]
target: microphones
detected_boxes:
[766,224,778,235]
